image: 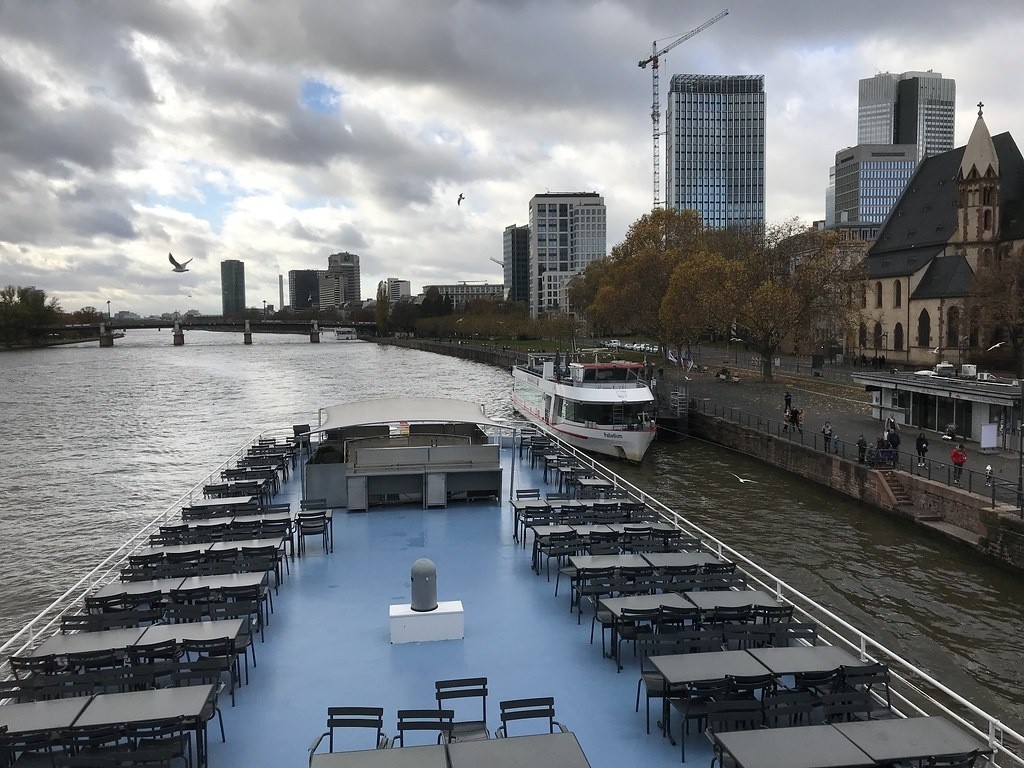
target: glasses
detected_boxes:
[921,436,924,437]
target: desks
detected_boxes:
[178,572,269,634]
[567,555,651,615]
[569,524,614,567]
[578,498,636,525]
[74,684,214,768]
[0,694,92,734]
[210,536,286,586]
[137,618,245,696]
[595,593,698,671]
[209,477,266,506]
[543,455,569,485]
[558,466,587,494]
[170,516,236,528]
[310,732,593,768]
[193,495,253,509]
[546,498,583,525]
[135,542,214,556]
[831,717,994,768]
[648,650,773,746]
[684,589,783,658]
[92,577,190,615]
[509,500,549,544]
[640,553,725,593]
[529,524,575,576]
[233,512,293,557]
[711,724,877,768]
[746,646,869,729]
[606,523,651,555]
[226,464,279,497]
[28,626,148,658]
[647,522,680,553]
[578,479,615,499]
[294,509,333,557]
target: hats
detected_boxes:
[889,429,895,433]
[959,444,965,448]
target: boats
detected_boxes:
[512,349,656,462]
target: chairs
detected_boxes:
[309,677,568,757]
[508,426,993,768]
[0,436,333,768]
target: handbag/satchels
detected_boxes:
[922,447,929,453]
[883,430,889,440]
[784,414,789,421]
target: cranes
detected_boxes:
[638,9,730,211]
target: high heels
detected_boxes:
[783,430,789,434]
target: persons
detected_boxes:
[640,366,653,380]
[985,464,993,487]
[951,444,968,484]
[855,434,867,464]
[866,429,902,470]
[784,391,792,411]
[658,365,664,380]
[854,352,886,371]
[447,338,546,355]
[716,366,730,384]
[916,432,928,467]
[834,435,840,453]
[783,406,805,434]
[821,421,832,454]
[885,413,900,433]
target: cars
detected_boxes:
[667,352,678,361]
[605,340,620,347]
[624,343,663,353]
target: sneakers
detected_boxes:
[918,463,924,467]
[986,483,992,486]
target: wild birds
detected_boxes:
[307,294,313,302]
[926,347,940,354]
[187,295,193,299]
[457,192,466,206]
[168,252,194,272]
[727,471,759,484]
[683,375,692,381]
[729,338,744,342]
[455,316,464,323]
[987,341,1006,352]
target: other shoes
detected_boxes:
[835,450,838,453]
[825,451,831,453]
[799,431,803,434]
[798,429,800,430]
[793,430,795,432]
[954,479,960,483]
